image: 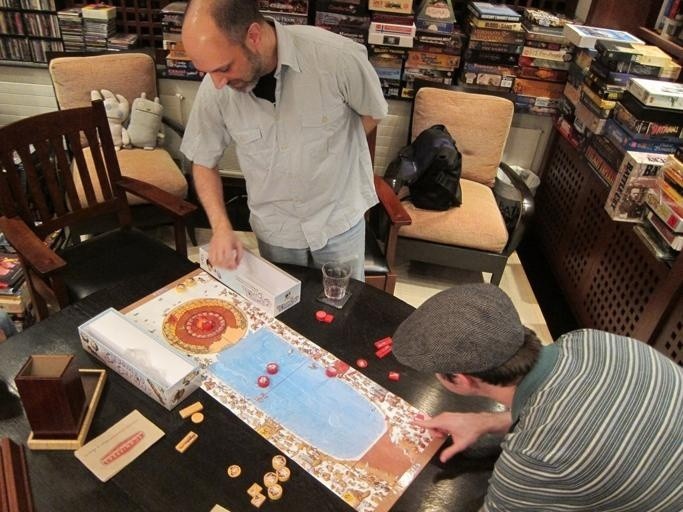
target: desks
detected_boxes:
[0,260,513,512]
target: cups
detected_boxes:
[321,261,353,300]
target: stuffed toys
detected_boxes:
[89,87,129,152]
[123,91,166,150]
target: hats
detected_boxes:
[392,283,526,374]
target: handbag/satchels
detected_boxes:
[383,123,462,211]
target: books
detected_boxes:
[555,0,682,260]
[160,0,575,115]
[1,0,138,64]
[0,225,73,333]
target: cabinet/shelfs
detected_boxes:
[0,0,65,70]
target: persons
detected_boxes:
[179,0,388,283]
[389,280,683,511]
[619,185,644,218]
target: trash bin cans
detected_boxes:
[493,165,541,234]
